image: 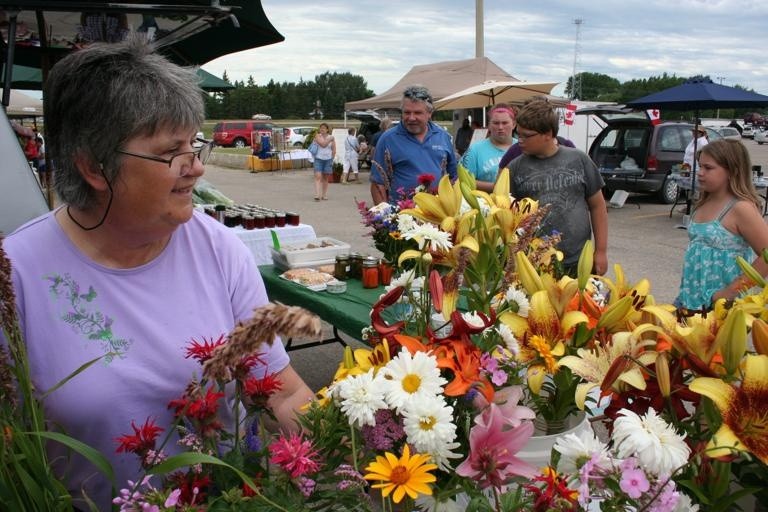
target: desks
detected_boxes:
[258,262,423,350]
[598,169,646,210]
[200,205,316,265]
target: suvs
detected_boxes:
[283,126,319,148]
[574,103,725,204]
[212,120,278,148]
[742,126,760,137]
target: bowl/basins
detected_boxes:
[327,281,347,293]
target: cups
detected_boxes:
[277,214,286,227]
[267,215,275,229]
[204,203,299,227]
[245,216,254,230]
[256,215,265,227]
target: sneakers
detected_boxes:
[678,206,687,212]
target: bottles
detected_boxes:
[362,262,379,288]
[380,258,393,285]
[204,203,299,230]
[349,254,358,277]
[335,255,350,279]
[356,255,366,280]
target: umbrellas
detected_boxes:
[431,77,561,108]
[623,82,768,196]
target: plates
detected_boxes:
[279,268,338,292]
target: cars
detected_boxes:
[251,113,271,120]
[708,126,742,142]
[754,130,768,145]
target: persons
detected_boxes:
[341,127,362,185]
[371,117,392,147]
[505,99,608,279]
[682,125,710,177]
[462,102,518,192]
[496,136,576,183]
[370,85,457,205]
[672,138,768,316]
[312,123,336,200]
[455,118,473,157]
[0,45,320,512]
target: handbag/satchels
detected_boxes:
[308,140,318,155]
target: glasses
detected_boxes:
[513,130,539,141]
[404,90,432,103]
[114,137,215,179]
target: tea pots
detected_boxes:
[213,205,224,227]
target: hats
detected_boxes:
[689,125,704,132]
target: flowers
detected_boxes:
[113,163,767,512]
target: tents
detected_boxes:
[345,55,519,127]
[0,1,287,217]
